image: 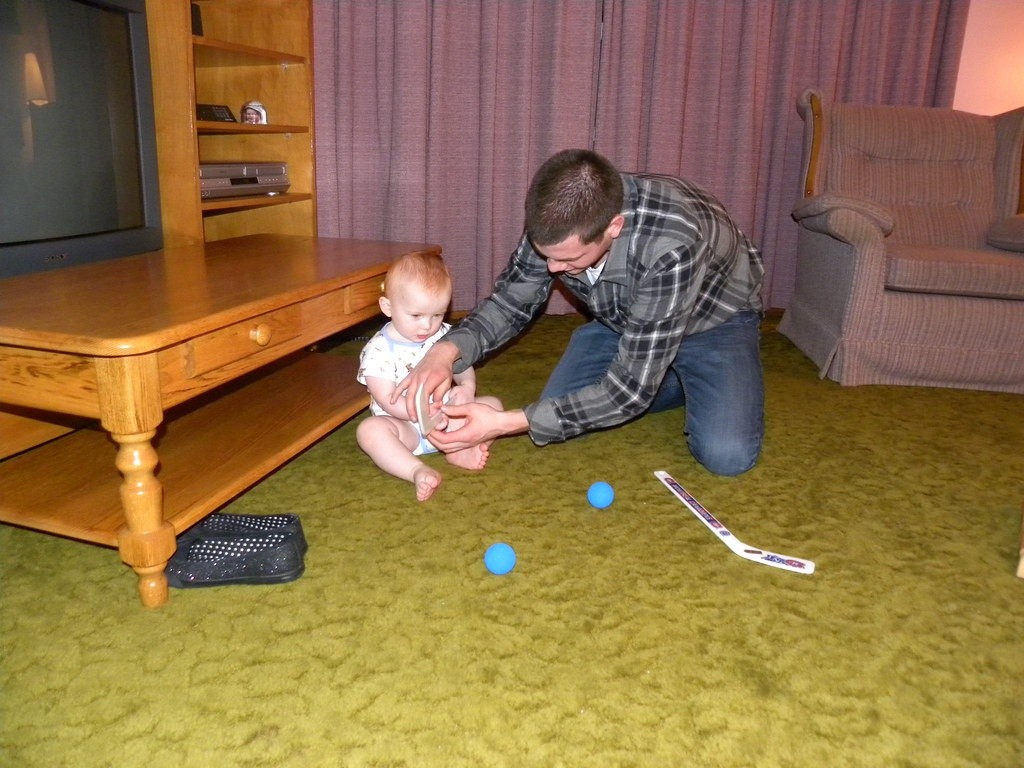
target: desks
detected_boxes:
[0,234,443,608]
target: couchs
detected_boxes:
[774,86,1024,395]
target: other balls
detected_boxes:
[586,480,616,510]
[483,542,519,576]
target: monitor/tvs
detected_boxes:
[0,0,163,278]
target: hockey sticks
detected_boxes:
[652,467,820,576]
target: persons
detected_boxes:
[356,251,504,501]
[242,109,261,124]
[391,149,763,476]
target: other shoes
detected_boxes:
[165,534,305,587]
[177,512,308,557]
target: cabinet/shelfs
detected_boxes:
[144,0,318,249]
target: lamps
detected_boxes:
[23,52,50,106]
